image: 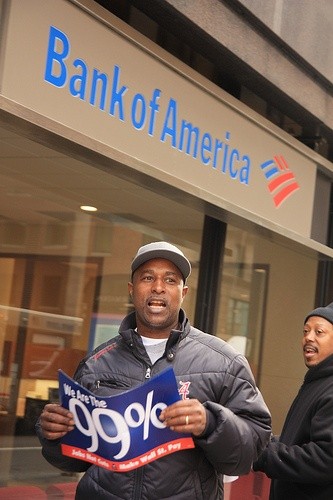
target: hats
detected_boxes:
[304,303,333,324]
[129,242,192,281]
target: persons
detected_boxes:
[36,238,274,500]
[252,302,333,500]
